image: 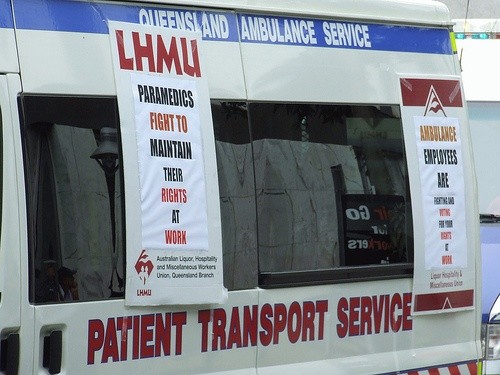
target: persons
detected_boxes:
[54,266,76,302]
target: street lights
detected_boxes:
[91,125,125,298]
